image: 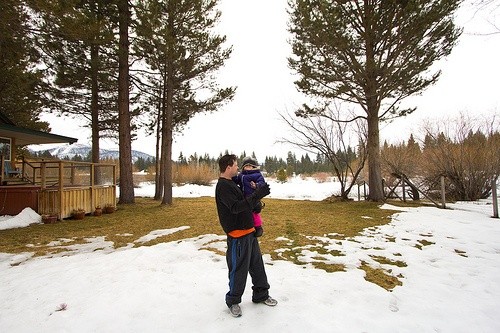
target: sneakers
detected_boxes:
[229,303,241,317]
[263,296,277,306]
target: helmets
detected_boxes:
[241,156,258,169]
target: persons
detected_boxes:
[214,154,278,318]
[231,155,270,238]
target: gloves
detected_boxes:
[256,183,270,198]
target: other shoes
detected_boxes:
[255,226,263,237]
